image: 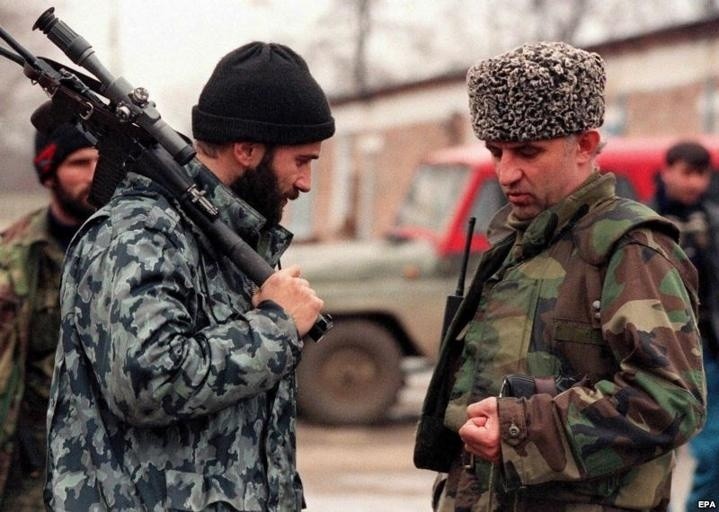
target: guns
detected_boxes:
[1,7,333,344]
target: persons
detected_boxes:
[43,41,334,512]
[0,96,98,511]
[413,42,710,512]
[645,141,718,511]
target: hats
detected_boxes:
[192,42,335,145]
[29,100,90,182]
[465,43,606,141]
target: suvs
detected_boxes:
[265,129,719,422]
